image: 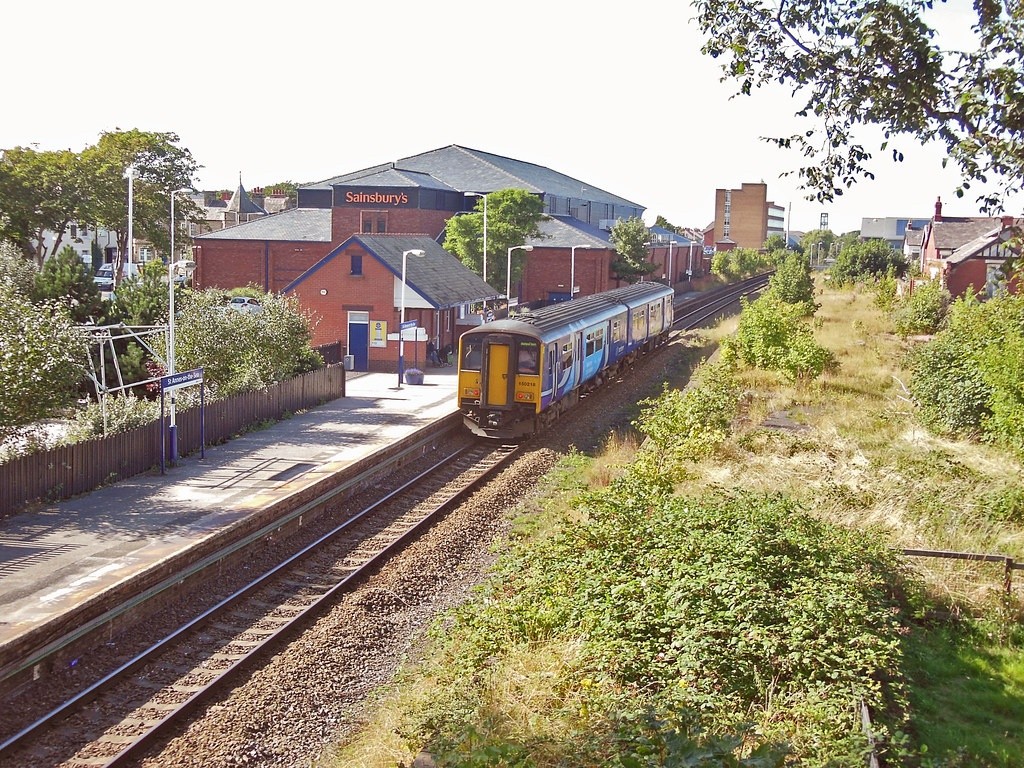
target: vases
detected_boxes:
[406,375,423,385]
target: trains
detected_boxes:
[457,281,675,439]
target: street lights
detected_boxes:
[399,248,427,383]
[169,187,194,468]
[125,164,141,279]
[570,244,591,300]
[688,241,697,283]
[506,245,533,300]
[810,242,844,266]
[464,191,487,283]
[668,241,678,288]
[641,242,650,281]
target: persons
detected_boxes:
[426,339,442,367]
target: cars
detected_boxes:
[173,260,196,277]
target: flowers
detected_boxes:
[405,368,424,375]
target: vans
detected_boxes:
[92,263,137,292]
